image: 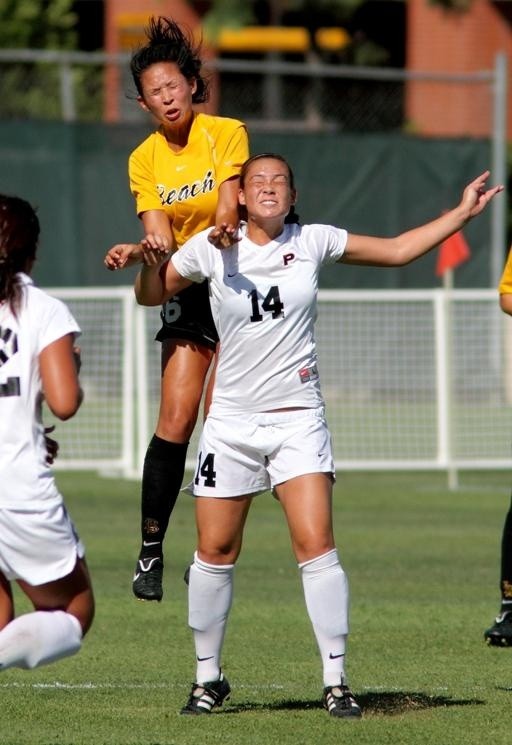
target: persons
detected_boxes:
[478,245,511,651]
[130,13,252,604]
[2,192,95,679]
[101,152,504,719]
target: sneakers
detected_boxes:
[179,666,232,717]
[130,543,166,603]
[482,607,512,648]
[320,684,363,720]
